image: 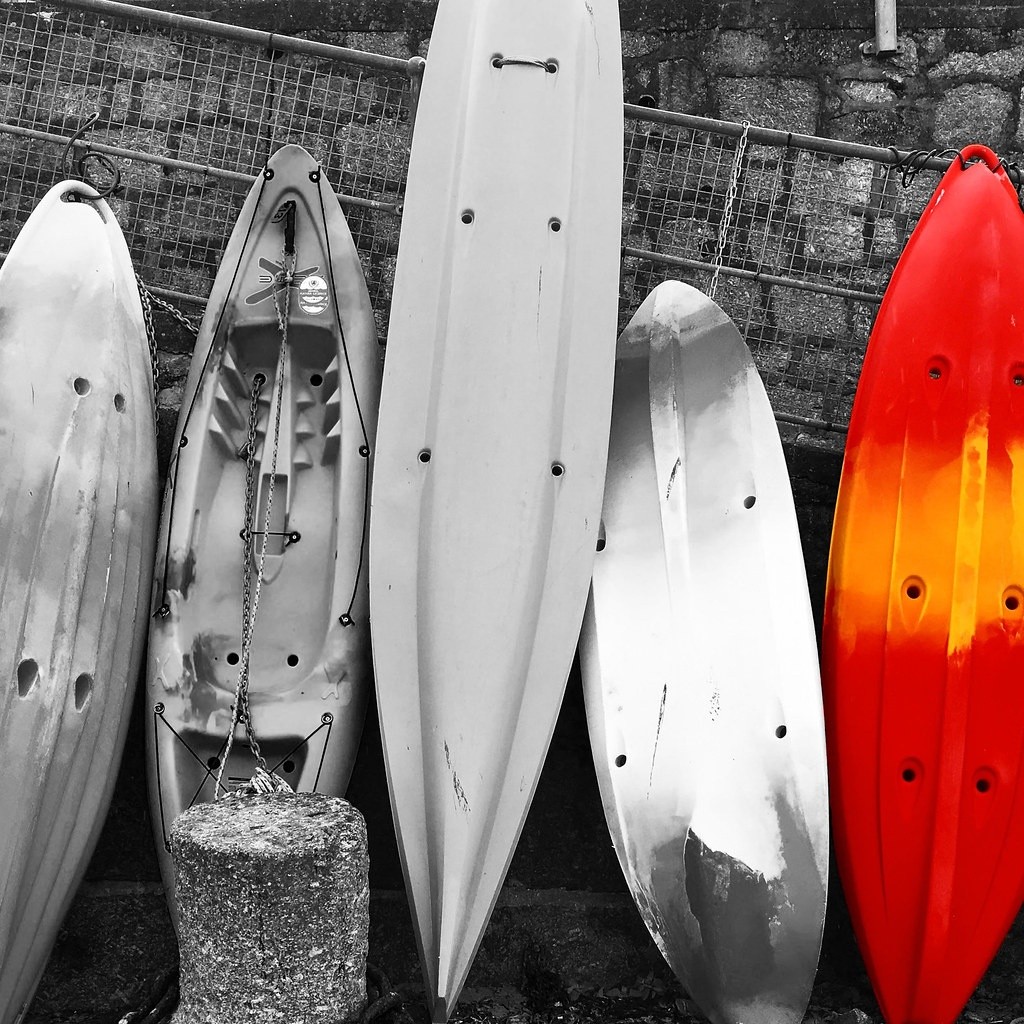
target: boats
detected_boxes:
[580,283,828,1024]
[369,1,624,1024]
[820,144,1024,1024]
[0,180,161,1024]
[142,146,372,946]
